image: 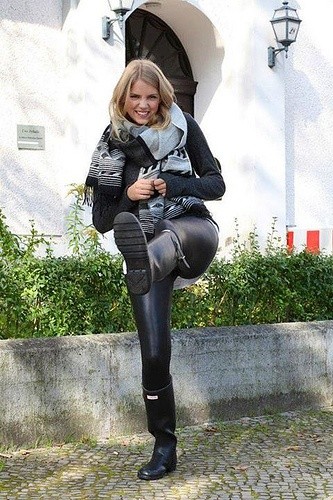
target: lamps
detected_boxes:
[102,0,135,40]
[268,0,302,68]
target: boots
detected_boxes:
[113,212,179,295]
[138,376,177,479]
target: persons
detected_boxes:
[85,58,227,481]
